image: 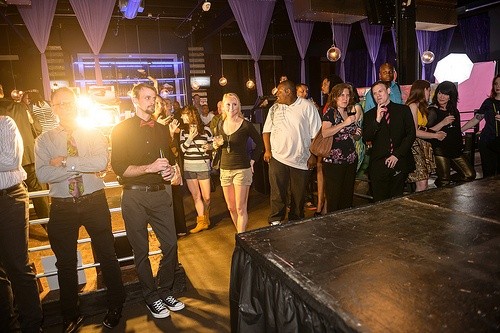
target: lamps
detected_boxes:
[420,47,435,64]
[272,41,278,95]
[328,18,341,62]
[193,28,255,91]
[120,0,144,19]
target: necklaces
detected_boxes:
[227,134,231,153]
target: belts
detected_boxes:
[51,188,105,203]
[127,184,165,192]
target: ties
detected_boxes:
[64,128,84,197]
[384,107,393,152]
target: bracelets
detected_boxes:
[62,157,67,167]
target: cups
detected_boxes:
[448,113,454,128]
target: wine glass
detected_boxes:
[348,106,357,127]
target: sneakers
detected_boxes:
[163,295,185,311]
[146,298,170,319]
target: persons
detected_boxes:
[462,75,500,177]
[0,63,477,333]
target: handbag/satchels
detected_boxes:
[209,149,221,176]
[418,125,438,144]
[309,125,333,158]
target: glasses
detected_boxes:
[53,101,75,105]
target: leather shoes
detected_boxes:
[59,314,84,332]
[103,308,122,328]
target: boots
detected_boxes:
[189,215,208,233]
[204,210,211,226]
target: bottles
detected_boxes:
[158,149,172,184]
